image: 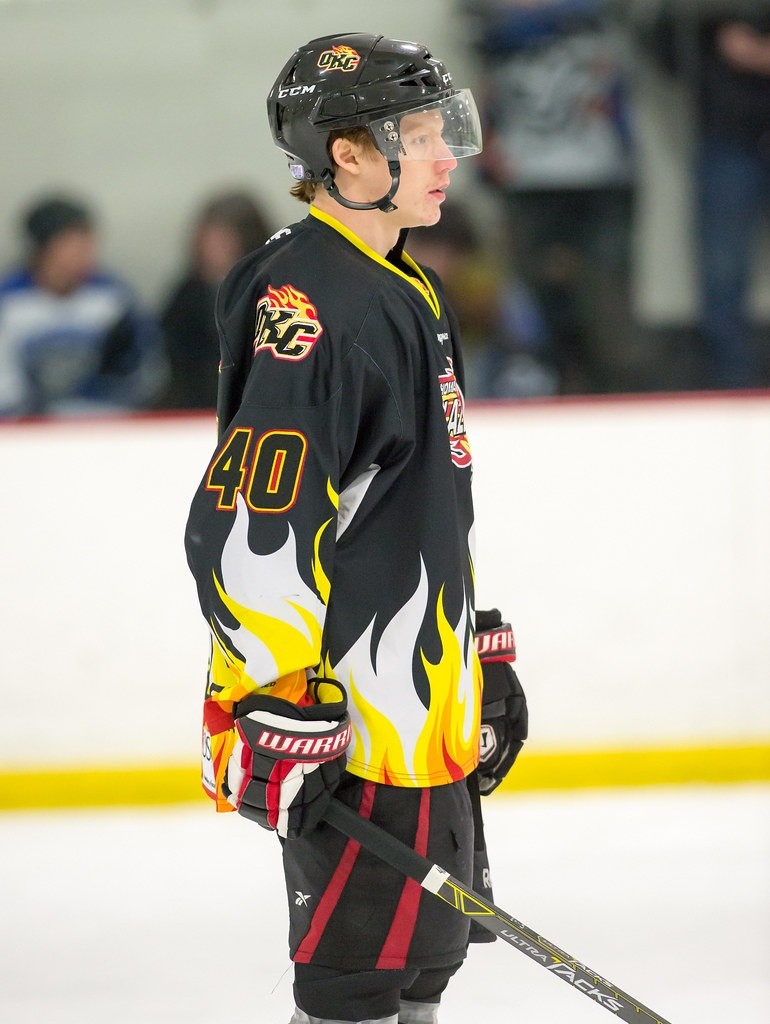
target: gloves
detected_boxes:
[219,678,354,844]
[468,607,529,795]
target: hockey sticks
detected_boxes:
[320,798,671,1024]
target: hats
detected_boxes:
[25,201,85,245]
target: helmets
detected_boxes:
[266,33,451,212]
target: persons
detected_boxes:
[184,32,529,1024]
[0,0,770,417]
